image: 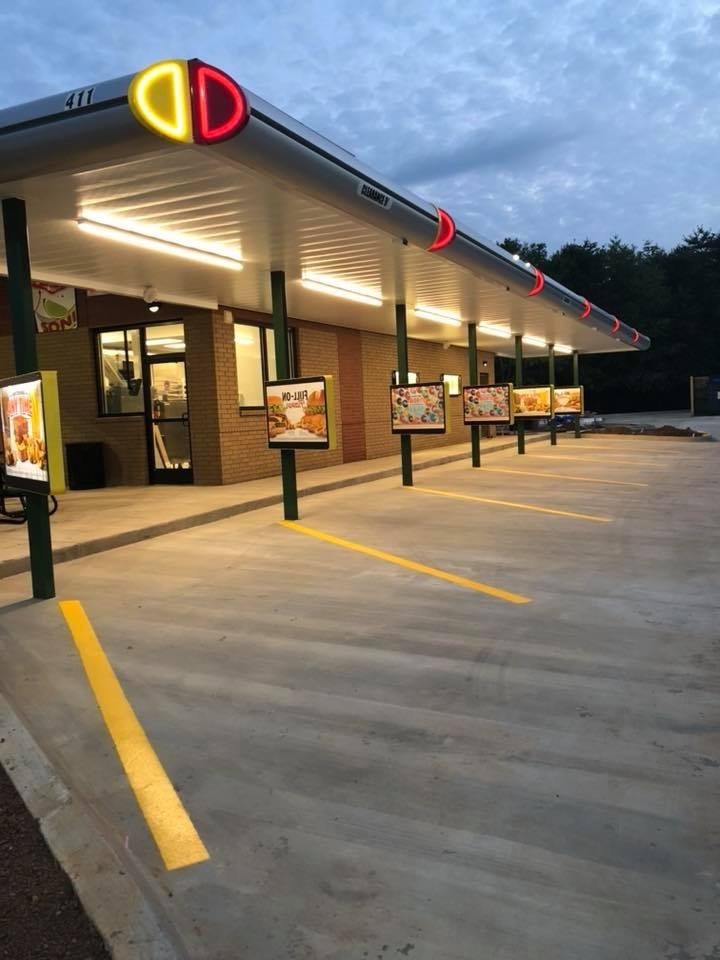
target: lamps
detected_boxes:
[80,218,574,358]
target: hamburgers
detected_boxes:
[300,390,328,437]
[266,396,286,436]
